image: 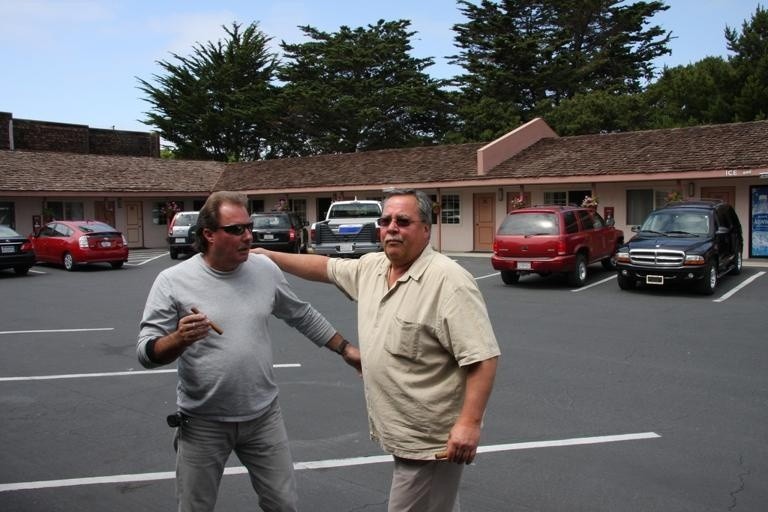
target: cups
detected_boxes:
[377,216,428,228]
[212,221,254,237]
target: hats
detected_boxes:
[0,226,35,277]
[27,220,128,270]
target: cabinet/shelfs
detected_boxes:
[752,194,768,255]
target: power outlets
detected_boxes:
[309,201,386,259]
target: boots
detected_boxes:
[166,210,202,261]
[490,206,626,287]
[615,196,745,297]
[249,211,310,254]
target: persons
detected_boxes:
[244,187,502,511]
[138,190,364,512]
